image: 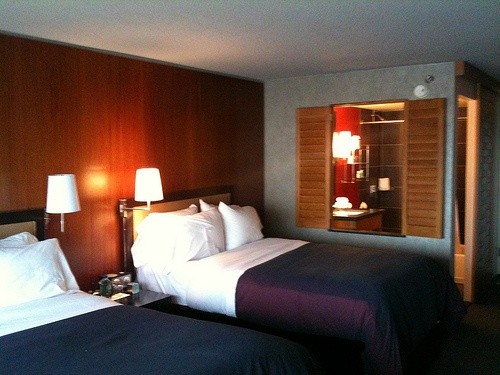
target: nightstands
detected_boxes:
[116,288,173,314]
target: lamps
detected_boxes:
[43,174,81,232]
[118,168,164,217]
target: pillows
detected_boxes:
[0,232,80,307]
[130,199,264,267]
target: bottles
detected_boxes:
[101,275,112,296]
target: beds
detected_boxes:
[120,185,467,375]
[0,206,316,375]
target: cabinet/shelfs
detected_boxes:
[330,117,467,231]
